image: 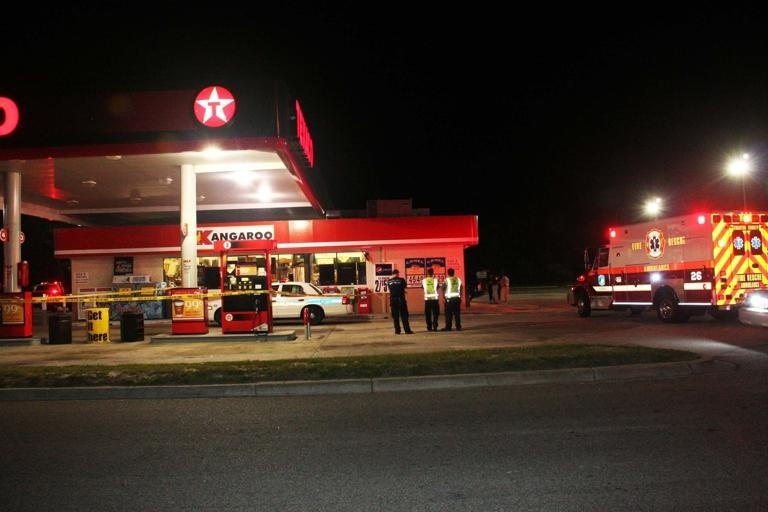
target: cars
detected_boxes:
[32,282,64,296]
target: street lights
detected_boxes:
[726,153,752,209]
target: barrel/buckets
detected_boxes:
[84,306,110,344]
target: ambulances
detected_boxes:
[567,209,768,323]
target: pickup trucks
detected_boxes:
[208,282,354,325]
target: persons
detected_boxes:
[486,270,511,305]
[421,268,440,332]
[440,268,463,331]
[387,269,413,335]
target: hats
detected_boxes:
[392,269,399,274]
